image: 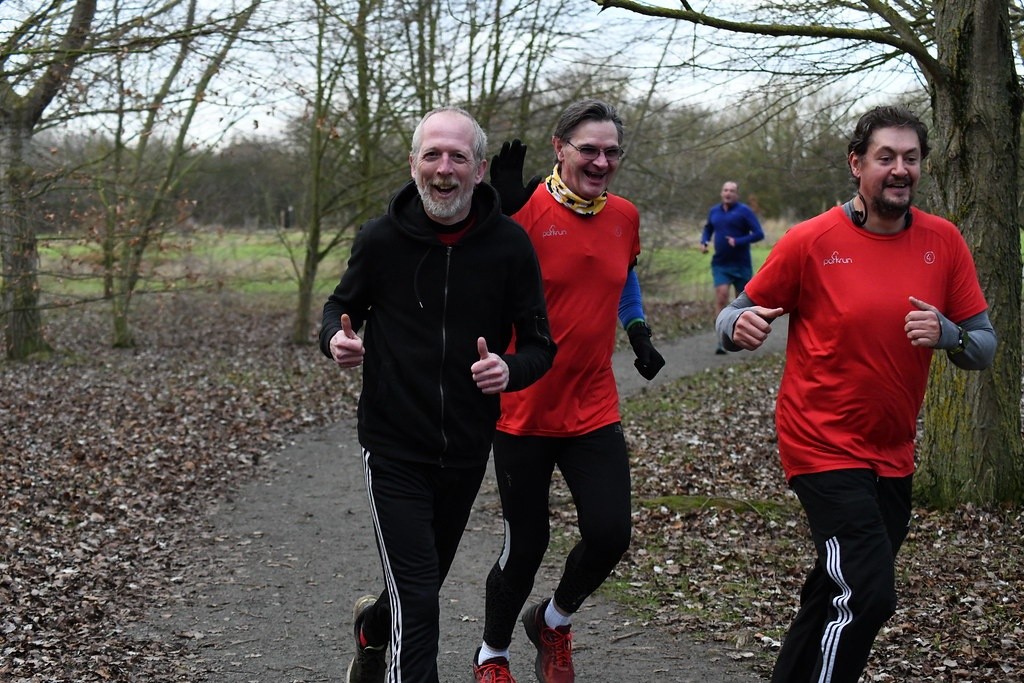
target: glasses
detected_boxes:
[558,135,624,161]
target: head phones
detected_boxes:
[849,189,913,231]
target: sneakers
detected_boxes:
[472,646,518,683]
[343,594,390,682]
[522,597,575,683]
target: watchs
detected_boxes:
[949,326,970,355]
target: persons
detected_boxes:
[320,101,557,683]
[700,182,765,354]
[472,99,666,683]
[716,107,997,683]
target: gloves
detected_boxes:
[490,137,543,217]
[627,321,665,381]
